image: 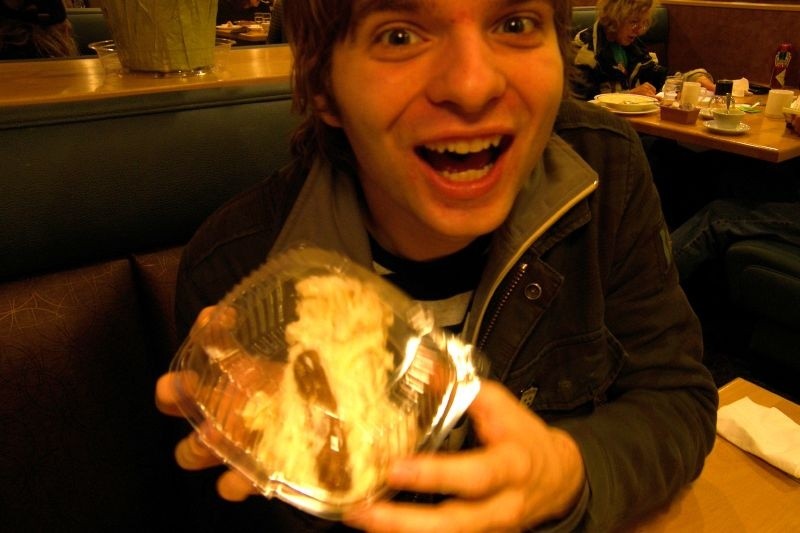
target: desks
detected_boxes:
[214,22,270,45]
[0,41,299,104]
[625,377,800,533]
[618,93,800,208]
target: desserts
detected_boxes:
[244,274,418,512]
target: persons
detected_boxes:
[568,0,678,101]
[0,0,81,60]
[155,0,720,533]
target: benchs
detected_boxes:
[0,76,313,531]
[572,6,670,51]
[723,238,800,395]
[63,7,114,52]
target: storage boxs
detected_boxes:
[660,103,702,124]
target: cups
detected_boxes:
[664,75,707,109]
[255,13,271,32]
[713,80,733,114]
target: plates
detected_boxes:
[587,93,751,136]
[216,21,264,32]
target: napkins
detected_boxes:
[716,397,800,481]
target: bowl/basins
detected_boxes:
[713,108,746,129]
[783,106,800,135]
